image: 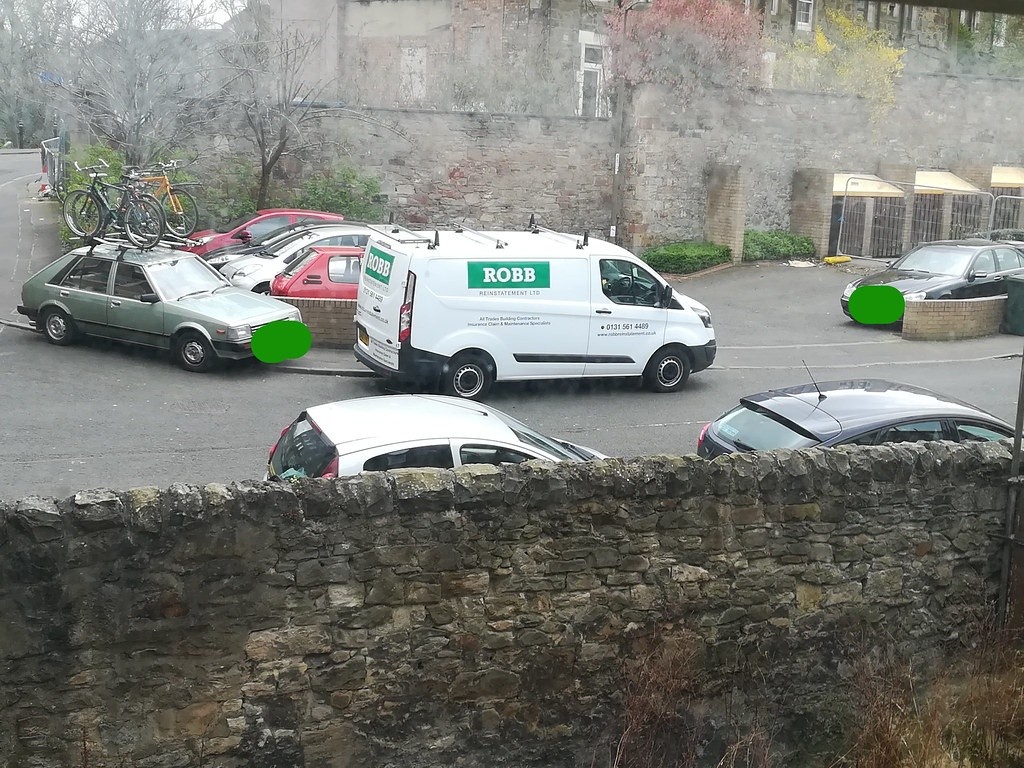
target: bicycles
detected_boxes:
[62,156,199,249]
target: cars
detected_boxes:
[200,220,373,299]
[840,239,1024,328]
[16,241,304,373]
[696,359,1024,462]
[172,206,344,256]
[260,392,612,485]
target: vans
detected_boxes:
[351,208,716,403]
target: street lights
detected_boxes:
[608,0,654,245]
